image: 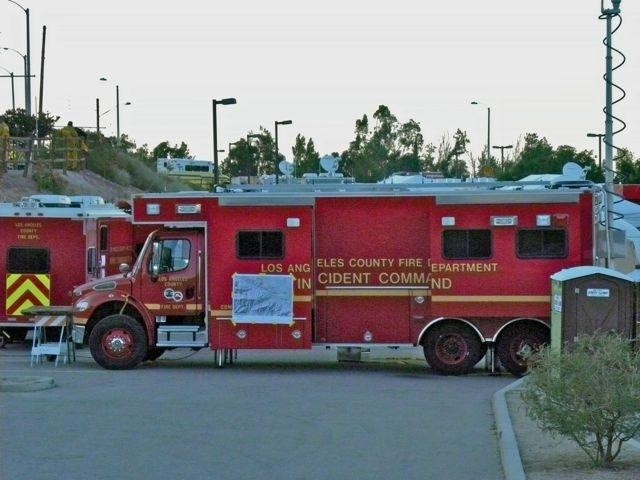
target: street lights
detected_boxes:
[100,77,120,138]
[212,98,237,184]
[2,45,31,120]
[493,145,513,173]
[471,101,491,159]
[274,120,293,184]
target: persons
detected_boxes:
[16,143,26,171]
[61,121,81,171]
[0,116,10,173]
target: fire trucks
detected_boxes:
[69,175,605,380]
[0,194,133,363]
